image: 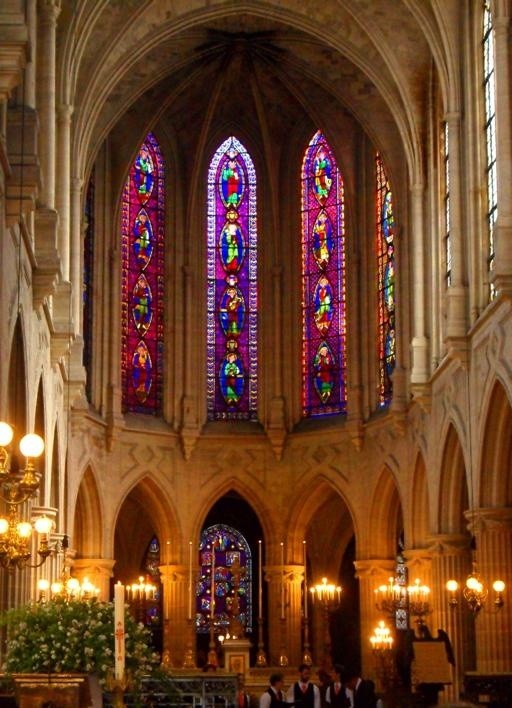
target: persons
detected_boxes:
[285,664,321,708]
[324,673,354,708]
[340,670,376,708]
[259,673,285,708]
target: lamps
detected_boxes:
[0,420,60,574]
[444,551,505,614]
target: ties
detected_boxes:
[334,682,339,692]
[301,684,305,691]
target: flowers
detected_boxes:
[0,591,173,707]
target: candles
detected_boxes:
[374,577,431,603]
[125,575,157,601]
[309,577,343,606]
